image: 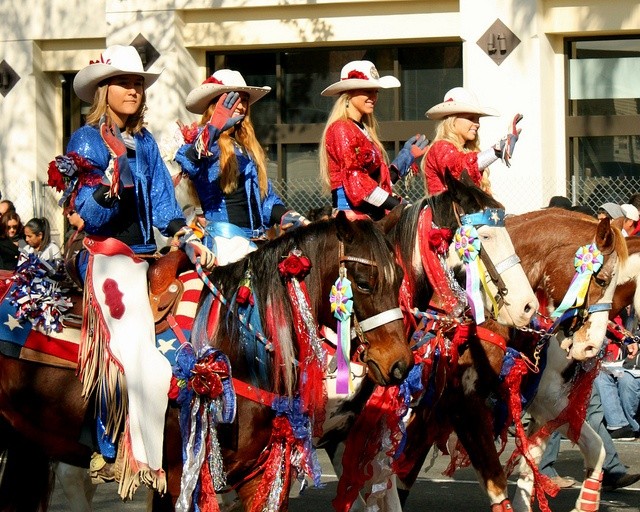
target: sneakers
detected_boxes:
[602,470,639,493]
[605,425,637,442]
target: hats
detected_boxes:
[185,69,271,114]
[73,44,166,106]
[425,87,501,121]
[619,204,640,222]
[597,202,624,220]
[320,59,401,96]
[540,196,572,210]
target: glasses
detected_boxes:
[69,211,77,216]
[5,224,19,230]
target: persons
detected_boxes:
[306,203,333,223]
[616,204,640,438]
[175,69,313,267]
[539,382,640,492]
[318,60,429,221]
[64,206,87,262]
[420,86,524,198]
[195,207,208,234]
[579,202,624,439]
[570,206,593,216]
[47,45,218,481]
[0,200,16,218]
[540,196,572,210]
[0,211,26,270]
[16,217,63,267]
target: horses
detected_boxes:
[0,200,417,512]
[323,165,541,512]
[395,206,630,512]
[510,235,640,512]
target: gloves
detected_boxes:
[385,198,409,216]
[389,134,429,178]
[494,113,523,167]
[194,91,246,157]
[170,224,217,272]
[279,210,310,231]
[99,112,134,189]
